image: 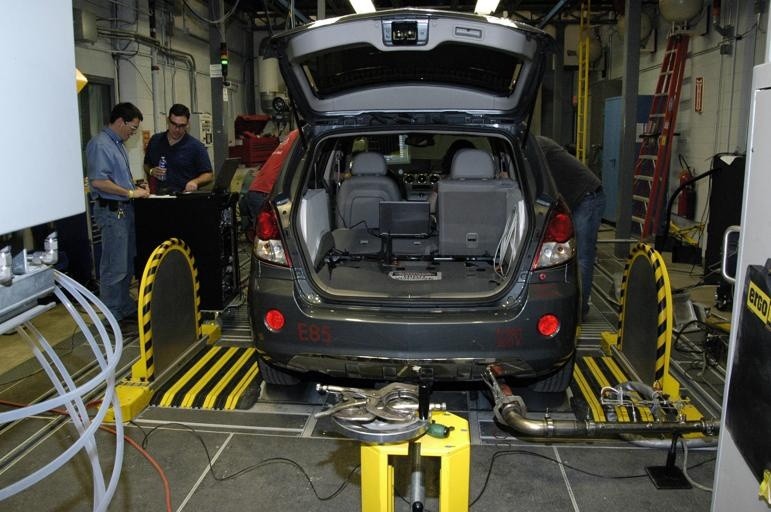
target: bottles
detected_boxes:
[157,157,167,182]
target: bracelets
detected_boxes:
[128,190,134,198]
[149,168,153,176]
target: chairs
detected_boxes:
[448,148,495,181]
[334,152,401,231]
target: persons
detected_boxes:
[144,104,213,195]
[536,135,606,318]
[86,101,150,336]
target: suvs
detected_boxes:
[247,7,581,394]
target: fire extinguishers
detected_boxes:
[676,151,695,219]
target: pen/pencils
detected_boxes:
[135,183,146,189]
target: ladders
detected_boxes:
[575,1,591,163]
[631,22,694,244]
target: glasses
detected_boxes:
[168,117,190,129]
[123,122,140,131]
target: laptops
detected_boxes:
[175,157,242,197]
